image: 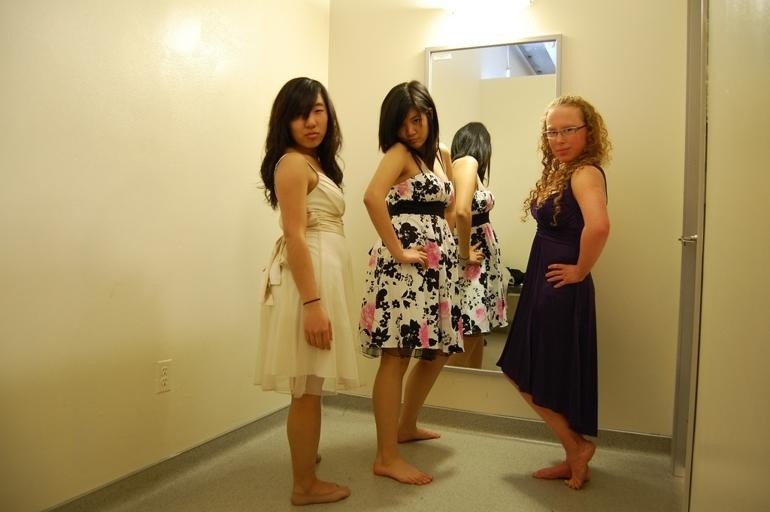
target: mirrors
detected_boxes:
[422,33,562,380]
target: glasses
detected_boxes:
[543,124,586,138]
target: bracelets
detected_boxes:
[302,298,322,305]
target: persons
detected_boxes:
[497,95,612,489]
[361,78,466,486]
[260,78,357,506]
[447,118,510,369]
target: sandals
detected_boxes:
[292,483,351,506]
